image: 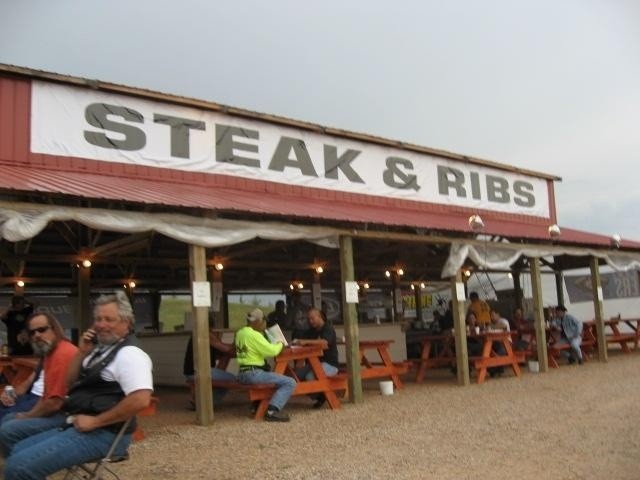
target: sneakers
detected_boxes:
[313,395,325,409]
[264,411,289,421]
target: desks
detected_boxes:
[1,356,40,390]
[340,340,404,403]
[218,345,340,420]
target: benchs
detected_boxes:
[359,362,414,369]
[411,320,638,384]
[187,379,274,408]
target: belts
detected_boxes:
[238,365,259,371]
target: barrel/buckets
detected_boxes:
[379,380,394,396]
[528,360,539,373]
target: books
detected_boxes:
[264,324,288,346]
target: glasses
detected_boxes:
[29,326,52,336]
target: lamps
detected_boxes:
[548,224,561,238]
[468,214,484,231]
[610,233,621,248]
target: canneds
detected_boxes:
[4,386,16,407]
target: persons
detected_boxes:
[286,290,315,339]
[235,308,296,422]
[1,350,44,418]
[1,295,37,356]
[0,309,78,455]
[182,320,235,411]
[433,301,583,378]
[0,290,154,479]
[294,308,339,407]
[467,291,491,325]
[265,300,287,330]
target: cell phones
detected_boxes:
[90,333,99,344]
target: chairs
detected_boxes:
[57,396,148,480]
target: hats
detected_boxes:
[248,309,264,321]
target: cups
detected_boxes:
[475,327,480,335]
[5,386,17,407]
